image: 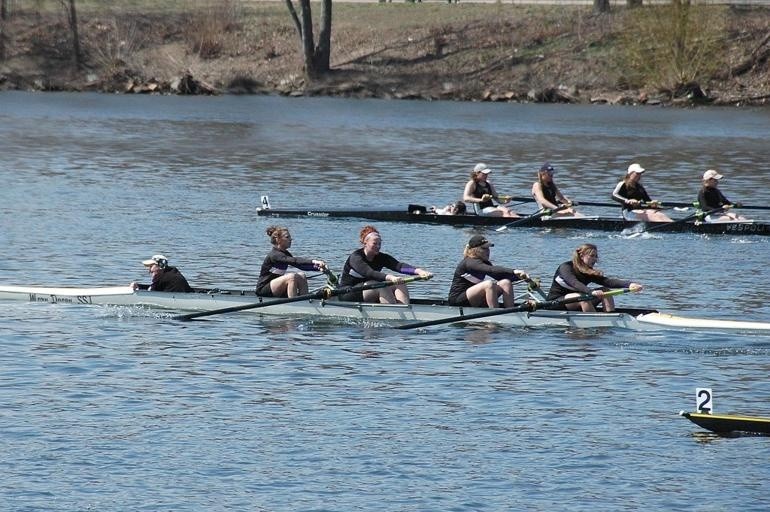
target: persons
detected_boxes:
[545,243,643,312]
[447,234,530,308]
[695,169,753,223]
[255,224,328,300]
[336,225,433,305]
[611,162,676,222]
[130,254,196,293]
[531,162,588,220]
[463,163,522,218]
[430,200,467,217]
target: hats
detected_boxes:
[469,236,494,249]
[703,170,724,180]
[474,163,492,174]
[540,165,553,173]
[627,163,645,175]
[142,255,168,269]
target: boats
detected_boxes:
[0,278,770,336]
[678,408,770,436]
[254,202,770,237]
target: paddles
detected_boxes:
[171,275,425,320]
[390,286,637,330]
